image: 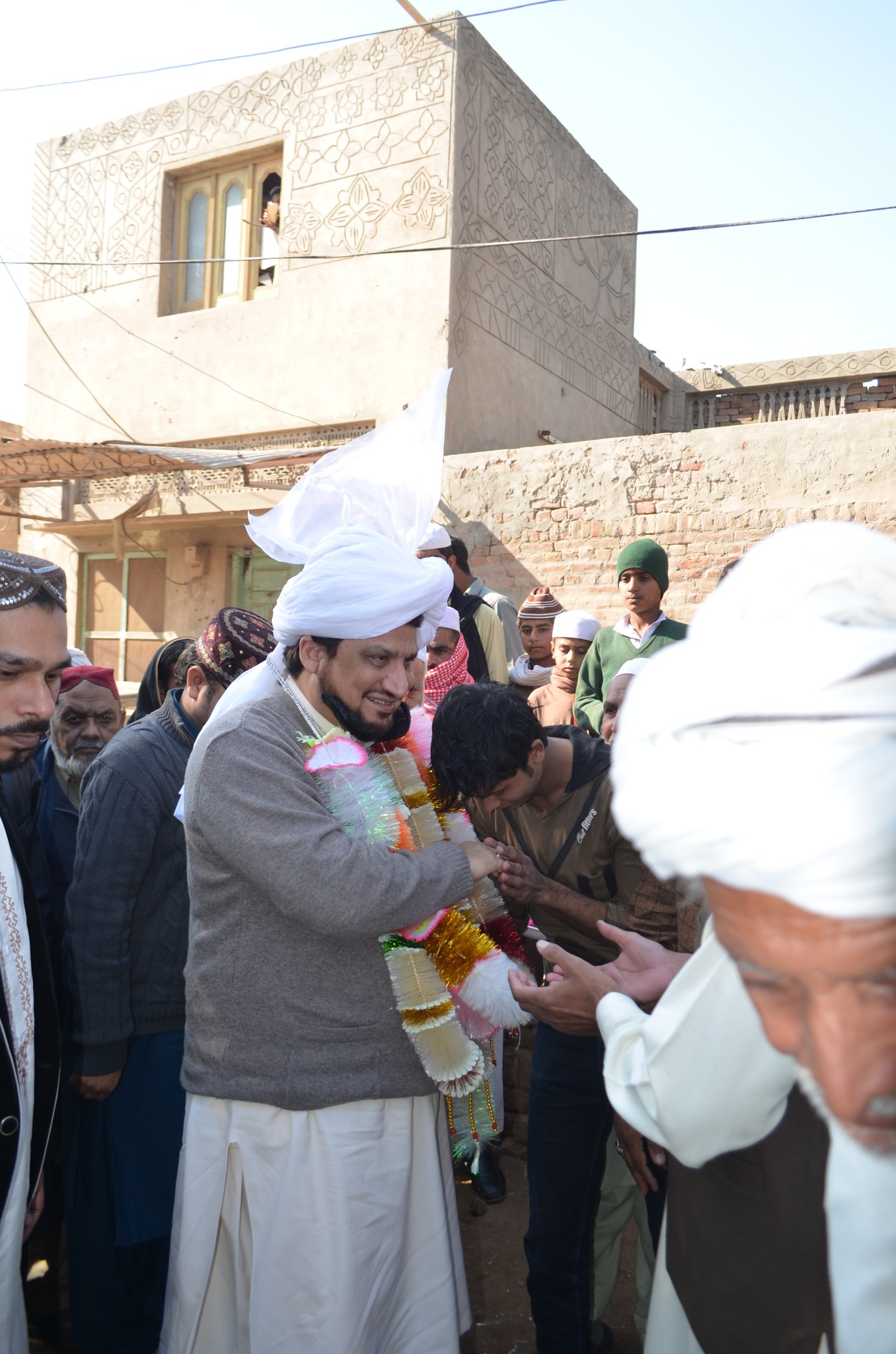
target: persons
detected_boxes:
[25,664,129,1347]
[506,516,896,1354]
[609,865,713,1257]
[257,182,281,287]
[0,548,76,1354]
[421,604,510,1207]
[61,606,278,1354]
[428,677,648,1354]
[3,536,746,1026]
[606,606,896,1354]
[156,538,514,1352]
[584,656,654,1354]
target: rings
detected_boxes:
[615,1140,620,1148]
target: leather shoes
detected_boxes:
[468,1145,507,1205]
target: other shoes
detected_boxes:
[590,1324,615,1354]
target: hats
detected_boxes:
[241,366,455,651]
[57,665,121,700]
[610,657,649,681]
[1,550,66,614]
[517,586,566,619]
[616,538,669,599]
[65,647,91,666]
[437,606,461,635]
[417,523,451,550]
[607,522,895,922]
[195,606,277,685]
[551,609,601,642]
